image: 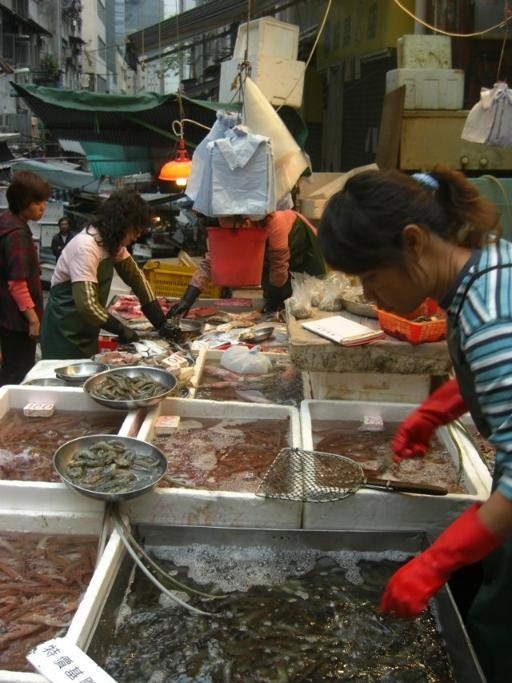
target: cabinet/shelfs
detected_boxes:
[398,109,512,172]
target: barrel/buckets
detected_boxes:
[207,227,270,289]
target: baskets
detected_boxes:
[370,298,448,346]
[146,260,220,298]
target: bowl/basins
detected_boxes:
[166,318,203,338]
[23,362,111,386]
[83,366,178,411]
[239,327,274,343]
[52,434,169,503]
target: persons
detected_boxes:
[316,163,512,621]
[0,171,52,385]
[165,209,327,319]
[51,217,75,263]
[38,188,180,360]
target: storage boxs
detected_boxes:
[0,296,500,680]
[218,16,465,111]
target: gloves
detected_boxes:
[100,311,139,344]
[377,502,504,621]
[166,284,202,319]
[262,287,289,312]
[391,378,470,465]
[141,299,181,339]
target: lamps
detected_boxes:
[158,139,193,187]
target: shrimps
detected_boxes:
[66,439,161,494]
[104,545,459,682]
[0,531,109,671]
[89,372,168,403]
